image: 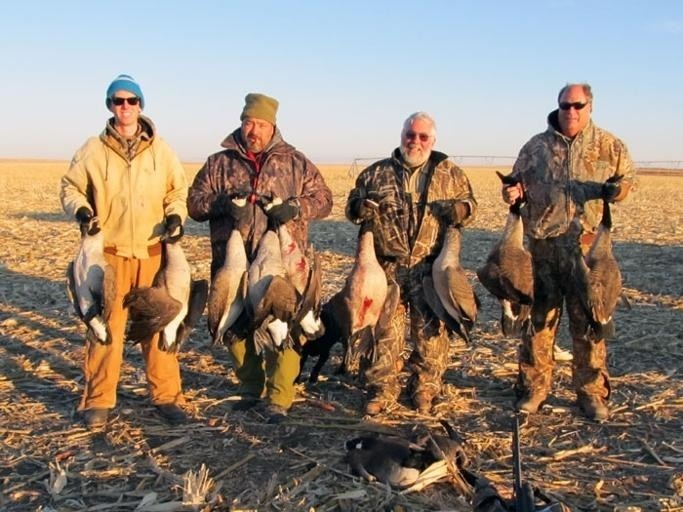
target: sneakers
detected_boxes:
[577,392,610,422]
[515,392,547,416]
[364,400,383,415]
[412,390,433,412]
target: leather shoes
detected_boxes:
[232,399,259,411]
[266,405,287,424]
[85,407,109,427]
[157,402,188,423]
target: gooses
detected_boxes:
[475,170,550,340]
[207,190,326,356]
[293,286,349,385]
[340,419,469,489]
[328,191,402,378]
[570,173,633,342]
[66,215,117,346]
[420,199,482,347]
[122,226,209,358]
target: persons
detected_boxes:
[186,92,332,425]
[58,72,192,430]
[342,110,479,418]
[499,82,634,421]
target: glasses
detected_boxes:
[111,96,140,106]
[406,132,429,141]
[559,102,589,109]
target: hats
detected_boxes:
[106,75,144,110]
[240,93,279,127]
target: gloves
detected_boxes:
[76,207,101,236]
[159,213,185,245]
[441,201,470,229]
[215,192,250,220]
[350,193,381,220]
[600,183,621,203]
[267,196,302,228]
